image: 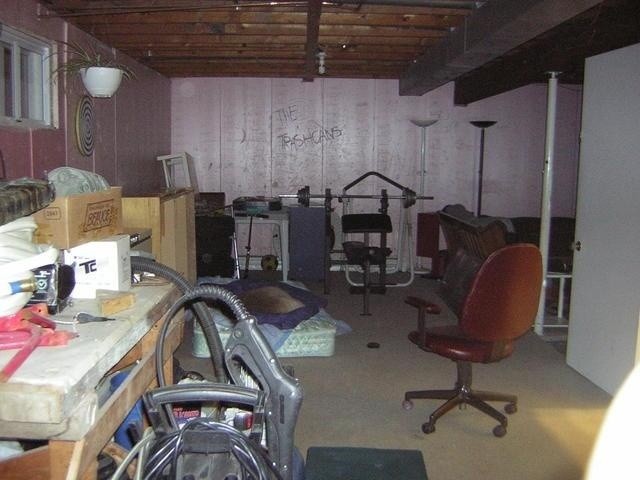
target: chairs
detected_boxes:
[402,243,543,436]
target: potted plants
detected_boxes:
[42,38,137,98]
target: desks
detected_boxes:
[0,281,186,480]
[532,271,572,328]
[224,206,289,280]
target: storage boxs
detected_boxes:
[64,234,132,300]
[33,186,121,250]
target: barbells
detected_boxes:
[279,186,433,208]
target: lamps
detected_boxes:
[470,121,496,218]
[408,119,438,275]
[318,52,327,75]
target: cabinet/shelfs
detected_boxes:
[121,191,198,290]
[122,227,153,253]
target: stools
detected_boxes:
[303,446,428,480]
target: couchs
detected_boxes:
[439,205,508,313]
[481,215,575,307]
